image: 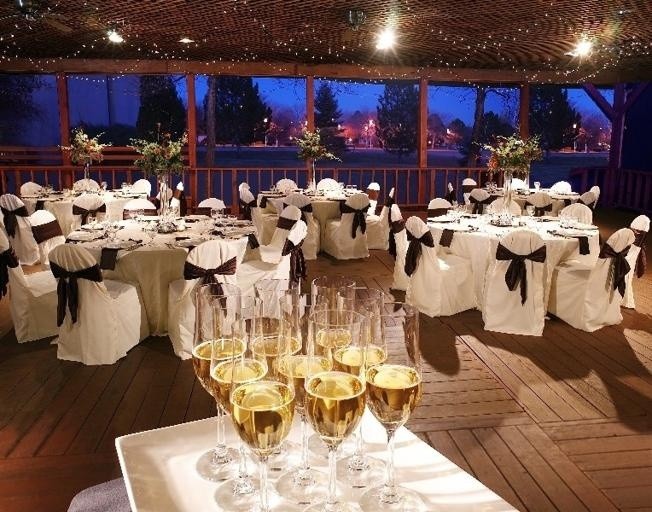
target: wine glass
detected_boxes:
[191,274,433,512]
[88,206,236,250]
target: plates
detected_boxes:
[426,211,478,233]
[533,214,599,239]
[167,229,257,248]
[66,231,137,249]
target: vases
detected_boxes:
[82,162,90,179]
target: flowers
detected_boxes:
[288,117,343,164]
[56,126,112,162]
[470,119,546,180]
[126,119,197,176]
